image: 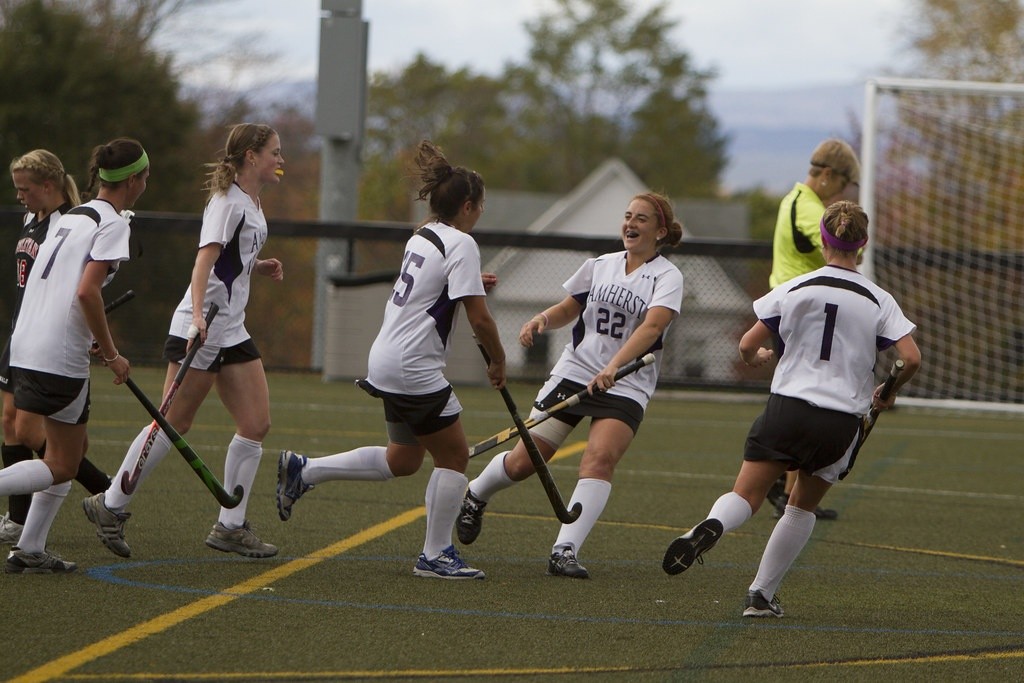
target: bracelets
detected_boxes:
[539,313,549,327]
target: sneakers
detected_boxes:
[742,589,786,618]
[82,493,131,557]
[662,518,724,576]
[546,547,589,578]
[275,450,315,521]
[0,512,23,544]
[456,484,488,546]
[205,520,279,558]
[413,545,485,580]
[6,547,76,574]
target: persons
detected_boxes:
[0,137,149,572]
[662,200,922,615]
[81,124,284,559]
[0,149,118,546]
[767,137,860,519]
[276,137,507,581]
[455,184,684,579]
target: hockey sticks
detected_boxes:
[103,289,135,313]
[118,301,221,496]
[467,351,657,458]
[92,341,245,510]
[472,332,584,525]
[838,358,906,483]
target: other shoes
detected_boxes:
[814,506,837,519]
